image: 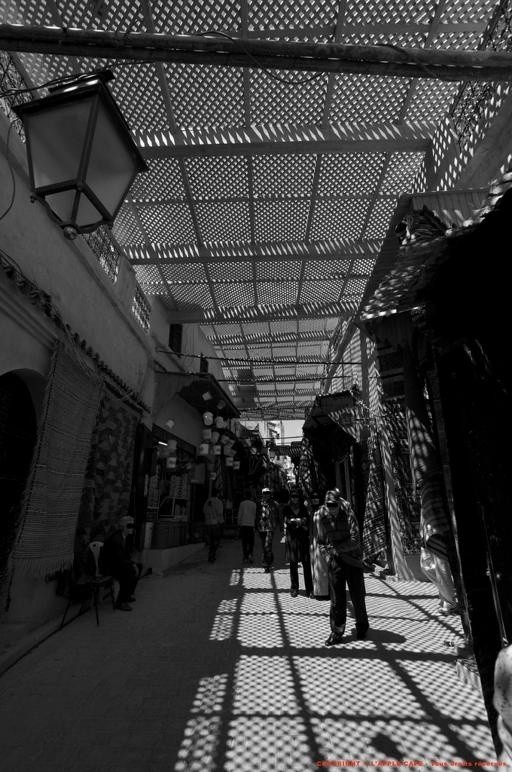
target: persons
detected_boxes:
[314,489,368,645]
[238,484,315,572]
[282,490,313,597]
[102,516,144,611]
[203,489,225,563]
[310,486,342,600]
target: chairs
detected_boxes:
[86,541,142,610]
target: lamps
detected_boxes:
[12,70,151,240]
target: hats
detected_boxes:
[261,488,270,494]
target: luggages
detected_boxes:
[312,545,330,597]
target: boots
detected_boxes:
[115,591,136,611]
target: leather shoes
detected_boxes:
[325,624,370,645]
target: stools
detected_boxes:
[58,575,116,629]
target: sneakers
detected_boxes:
[438,604,458,613]
[205,557,273,573]
[289,587,312,598]
